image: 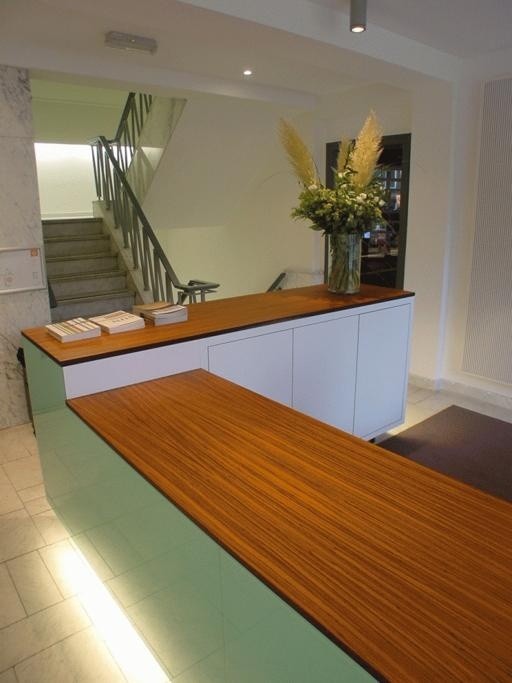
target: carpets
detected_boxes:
[376,403,512,503]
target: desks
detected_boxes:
[20,282,512,683]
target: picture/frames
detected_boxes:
[324,133,412,291]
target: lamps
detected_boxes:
[347,1,369,33]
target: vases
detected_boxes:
[325,232,363,296]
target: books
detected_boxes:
[46,316,103,344]
[89,308,145,334]
[132,300,188,327]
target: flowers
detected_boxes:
[272,108,394,236]
